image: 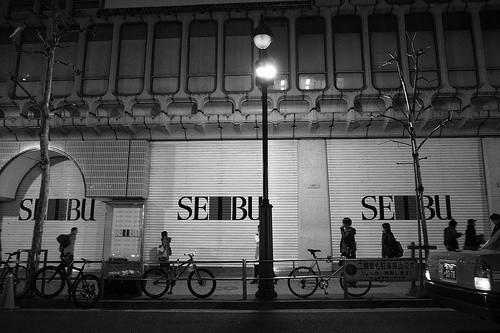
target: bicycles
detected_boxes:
[0,251,33,299]
[31,252,102,309]
[140,253,217,298]
[287,249,371,299]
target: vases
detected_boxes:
[104,279,147,300]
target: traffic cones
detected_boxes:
[0,278,21,310]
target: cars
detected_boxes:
[423,229,500,325]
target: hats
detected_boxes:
[468,219,477,224]
[490,213,500,220]
[449,220,457,226]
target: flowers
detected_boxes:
[105,270,143,279]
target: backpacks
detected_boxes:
[392,241,403,258]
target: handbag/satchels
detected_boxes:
[158,245,164,254]
[159,250,167,261]
[338,252,356,266]
[56,233,74,248]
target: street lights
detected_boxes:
[250,11,278,300]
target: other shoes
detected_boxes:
[273,276,280,284]
[250,279,258,284]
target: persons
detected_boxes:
[464,219,480,251]
[157,231,170,269]
[250,225,279,285]
[381,223,396,259]
[339,218,357,287]
[490,213,500,237]
[60,228,78,278]
[445,220,461,252]
